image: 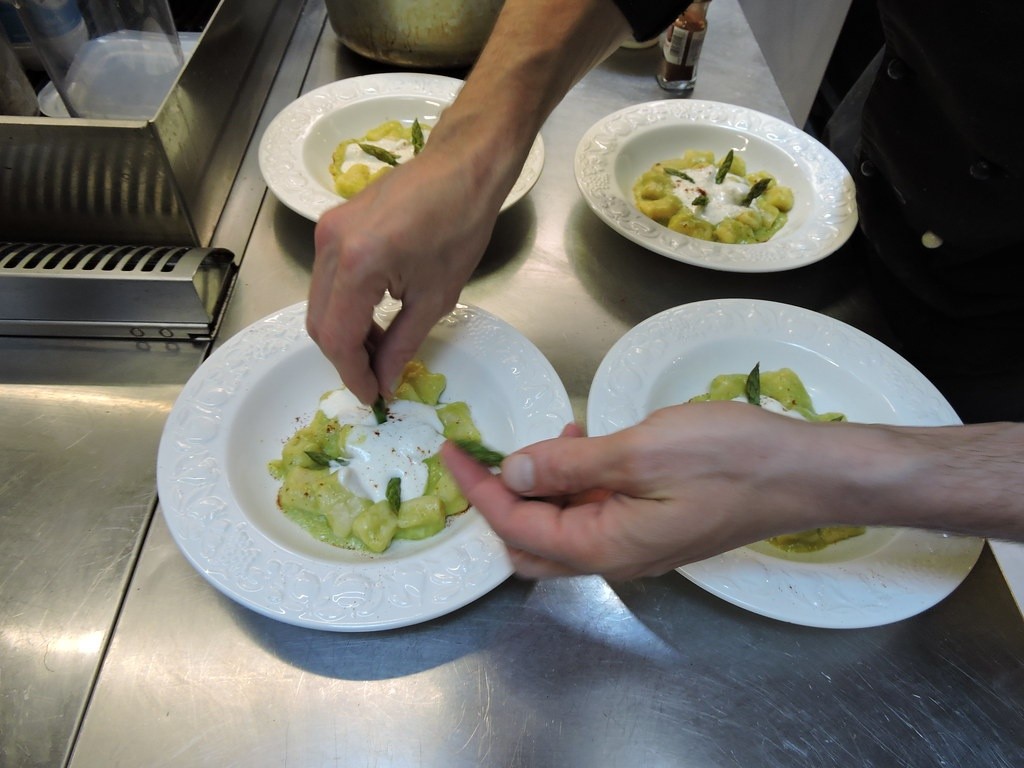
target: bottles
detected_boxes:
[654,0,713,89]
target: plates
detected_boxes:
[260,72,544,224]
[573,99,857,272]
[588,298,986,630]
[158,298,576,632]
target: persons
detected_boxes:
[304,0,1024,582]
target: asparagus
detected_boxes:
[663,149,772,207]
[302,393,505,518]
[744,362,762,405]
[357,117,423,167]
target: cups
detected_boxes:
[13,0,182,120]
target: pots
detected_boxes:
[325,0,505,68]
